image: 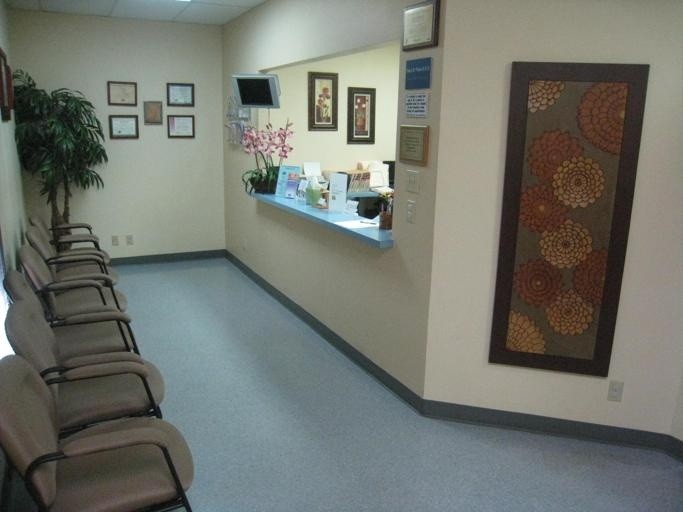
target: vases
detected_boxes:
[250,177,277,193]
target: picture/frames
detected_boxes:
[488,62,650,377]
[347,87,375,144]
[401,0,440,51]
[109,115,138,138]
[144,101,162,123]
[308,72,338,131]
[399,125,428,167]
[167,83,194,106]
[168,115,194,138]
[107,81,137,106]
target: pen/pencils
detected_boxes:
[360,220,376,225]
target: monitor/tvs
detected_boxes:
[230,75,281,109]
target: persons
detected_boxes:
[323,88,330,99]
[316,94,330,122]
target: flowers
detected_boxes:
[241,118,294,195]
